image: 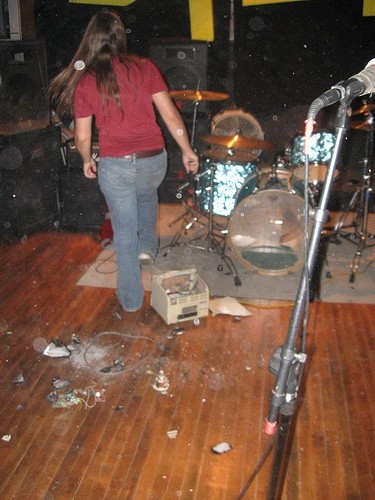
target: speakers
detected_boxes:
[149,39,229,202]
[0,38,105,239]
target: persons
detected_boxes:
[47,11,200,314]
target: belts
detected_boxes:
[123,148,163,159]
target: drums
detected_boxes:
[227,169,315,276]
[292,132,341,184]
[211,109,263,157]
[196,149,259,217]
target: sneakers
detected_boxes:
[137,252,154,264]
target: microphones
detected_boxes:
[307,59,375,122]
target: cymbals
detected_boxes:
[349,104,375,131]
[169,90,230,101]
[202,134,274,150]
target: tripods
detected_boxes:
[164,102,375,286]
